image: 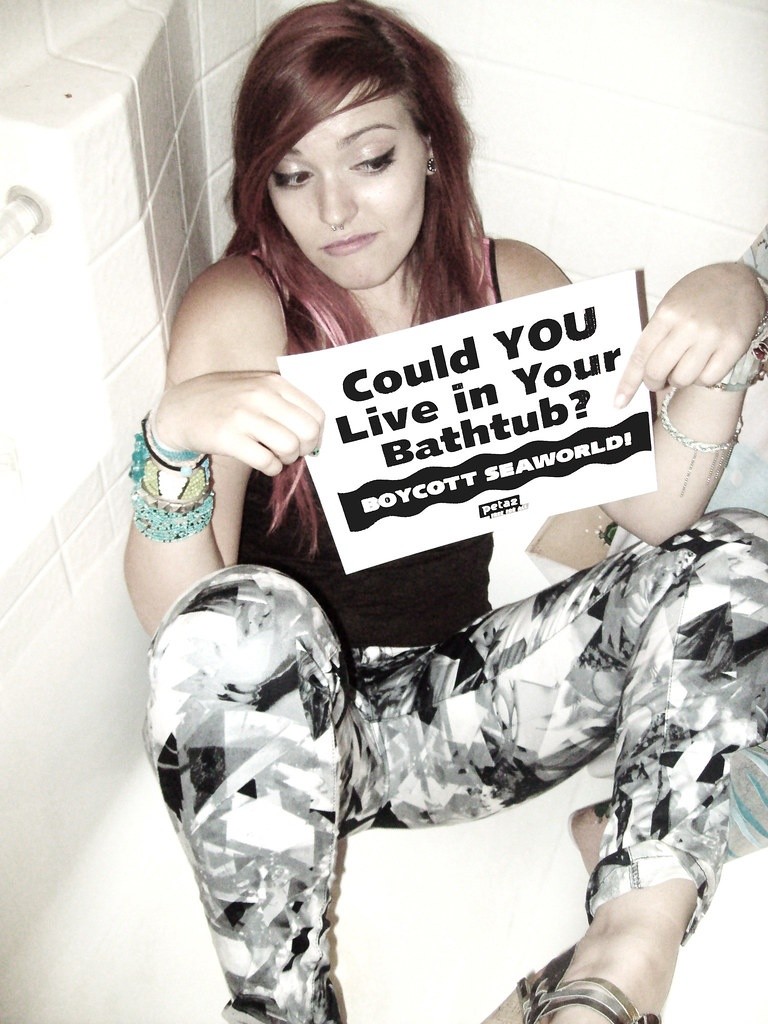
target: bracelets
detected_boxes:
[127,398,215,544]
[706,313,768,392]
[661,387,744,498]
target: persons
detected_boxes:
[122,1,768,1024]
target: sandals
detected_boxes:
[220,984,342,1024]
[516,944,662,1024]
